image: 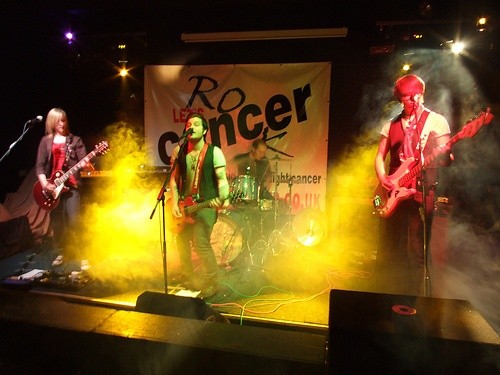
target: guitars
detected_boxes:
[32,140,112,211]
[370,109,494,217]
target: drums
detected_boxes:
[193,208,248,266]
[252,200,278,213]
[227,176,256,204]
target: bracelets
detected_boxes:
[44,182,50,190]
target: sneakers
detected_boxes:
[53,255,63,266]
[81,260,91,271]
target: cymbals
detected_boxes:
[281,178,301,183]
[259,157,295,161]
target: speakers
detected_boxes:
[135,291,230,325]
[325,288,500,375]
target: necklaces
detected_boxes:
[188,153,198,170]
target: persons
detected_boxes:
[170,114,229,299]
[36,108,91,271]
[233,139,275,207]
[374,76,453,296]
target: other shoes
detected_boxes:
[171,273,191,283]
[200,285,218,297]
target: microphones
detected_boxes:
[180,129,194,140]
[27,115,43,124]
[410,95,418,102]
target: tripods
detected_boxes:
[240,159,301,270]
